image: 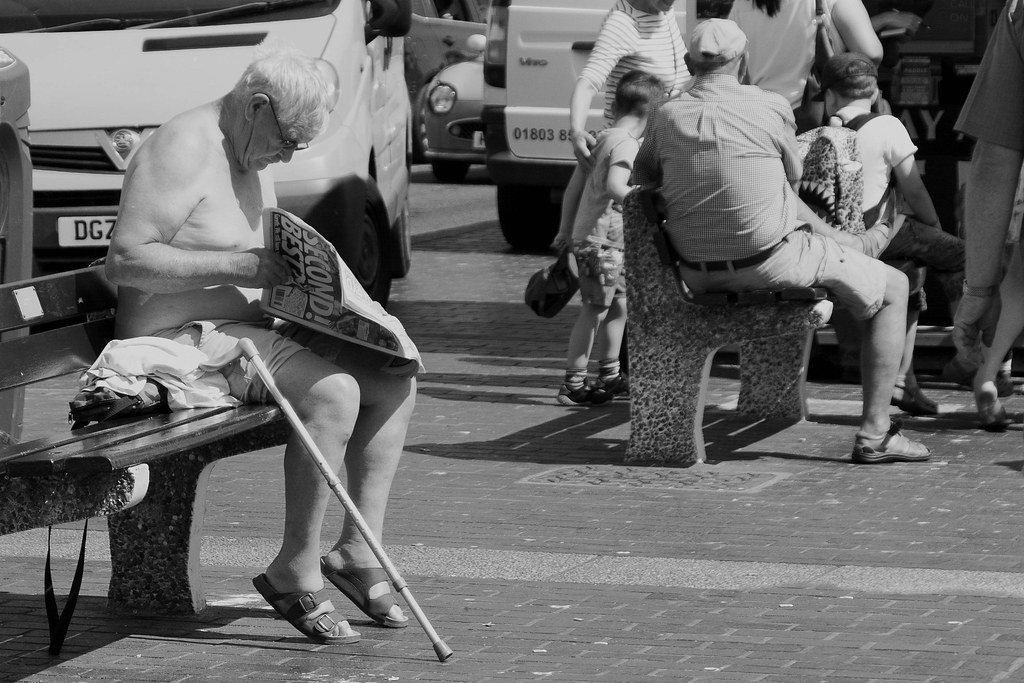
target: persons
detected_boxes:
[812,53,1013,414]
[628,17,930,465]
[570,0,692,170]
[951,0,1024,424]
[727,0,884,119]
[105,49,418,645]
[552,71,664,406]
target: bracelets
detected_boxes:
[963,278,999,297]
[930,217,939,226]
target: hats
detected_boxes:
[688,18,749,64]
[811,51,878,104]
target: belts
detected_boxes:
[681,240,780,272]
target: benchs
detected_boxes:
[0,264,291,624]
[621,183,837,468]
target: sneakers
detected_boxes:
[596,371,633,400]
[557,382,613,407]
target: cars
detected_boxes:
[415,34,486,182]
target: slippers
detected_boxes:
[979,411,1016,429]
[252,571,362,645]
[319,555,409,628]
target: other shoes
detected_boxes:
[943,355,1014,397]
[890,378,938,415]
[854,417,932,463]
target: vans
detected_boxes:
[483,0,735,250]
[404,0,492,108]
[0,0,413,311]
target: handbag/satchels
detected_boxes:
[69,376,161,425]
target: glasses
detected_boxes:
[253,90,309,152]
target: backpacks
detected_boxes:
[795,111,884,234]
